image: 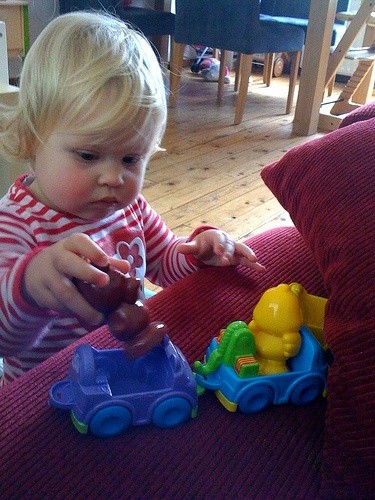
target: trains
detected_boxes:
[48,317,332,436]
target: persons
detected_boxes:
[0,11,266,387]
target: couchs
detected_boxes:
[0,103,375,500]
[260,116,375,500]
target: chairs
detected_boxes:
[59,0,351,125]
